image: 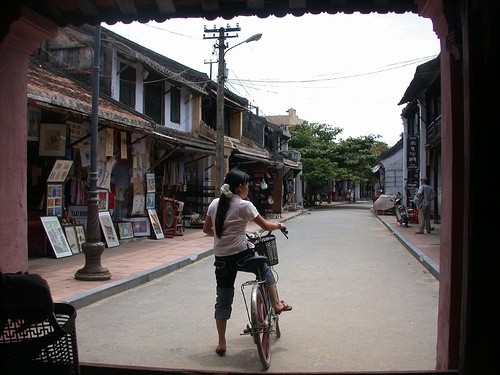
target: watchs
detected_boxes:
[276,221,281,228]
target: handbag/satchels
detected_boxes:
[413,186,424,206]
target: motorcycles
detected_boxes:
[390,192,410,228]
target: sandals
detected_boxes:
[216,346,226,353]
[275,301,289,314]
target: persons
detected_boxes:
[202,168,290,353]
[414,176,436,234]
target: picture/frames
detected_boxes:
[24,105,165,259]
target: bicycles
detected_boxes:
[0,271,80,375]
[240,223,289,369]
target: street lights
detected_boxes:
[214,32,262,197]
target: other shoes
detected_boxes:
[415,229,431,234]
